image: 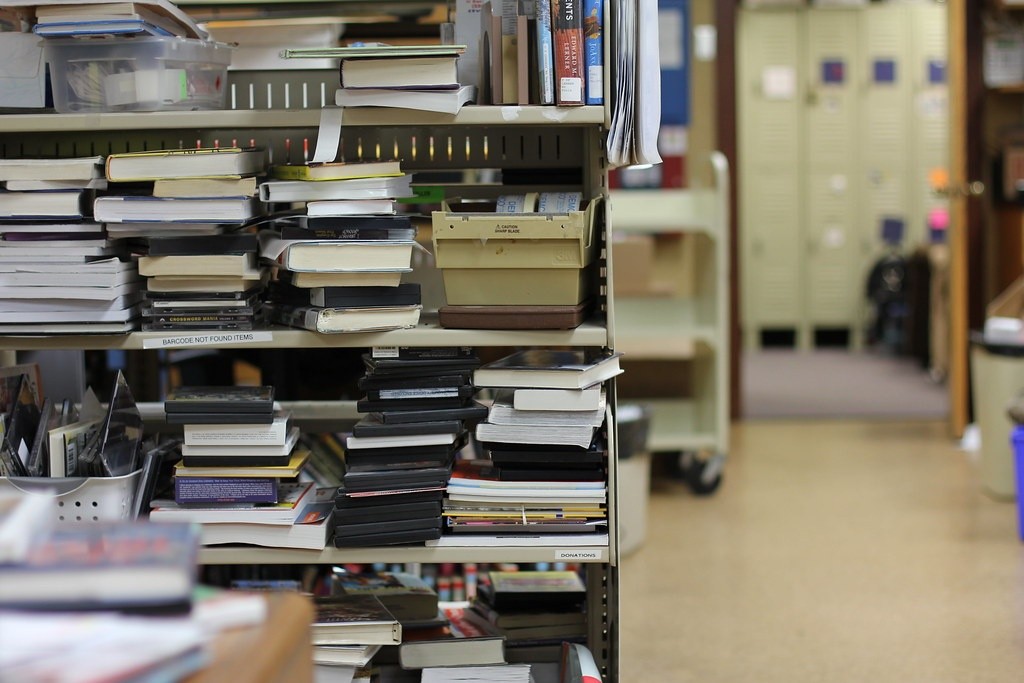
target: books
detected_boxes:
[0,0,690,683]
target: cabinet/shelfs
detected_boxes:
[0,0,622,683]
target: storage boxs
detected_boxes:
[0,466,143,524]
[432,193,603,305]
[0,32,47,108]
[36,38,237,113]
[615,404,652,550]
